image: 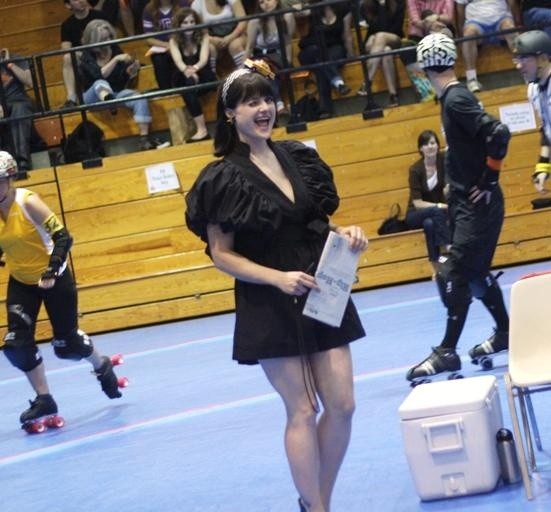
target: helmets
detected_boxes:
[0,150,19,179]
[511,29,551,57]
[415,32,459,69]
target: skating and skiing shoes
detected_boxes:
[404,345,465,387]
[19,393,65,434]
[92,353,130,400]
[468,330,508,369]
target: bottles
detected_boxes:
[492,427,524,486]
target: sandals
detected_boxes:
[185,133,211,143]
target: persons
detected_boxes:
[1,47,34,171]
[184,56,368,512]
[406,34,507,388]
[511,29,551,192]
[403,129,452,285]
[1,151,130,434]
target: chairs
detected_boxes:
[504,268,549,500]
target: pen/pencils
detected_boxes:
[288,261,314,299]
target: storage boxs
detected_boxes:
[400,374,504,506]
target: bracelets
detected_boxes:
[7,62,13,68]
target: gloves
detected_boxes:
[476,175,498,193]
[532,155,551,184]
[40,263,61,283]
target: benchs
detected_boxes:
[0,1,549,143]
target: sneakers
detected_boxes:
[337,83,351,97]
[387,93,401,108]
[277,102,288,117]
[18,159,32,171]
[58,99,78,108]
[139,137,170,151]
[318,111,334,120]
[467,78,483,93]
[104,92,118,116]
[356,79,373,97]
